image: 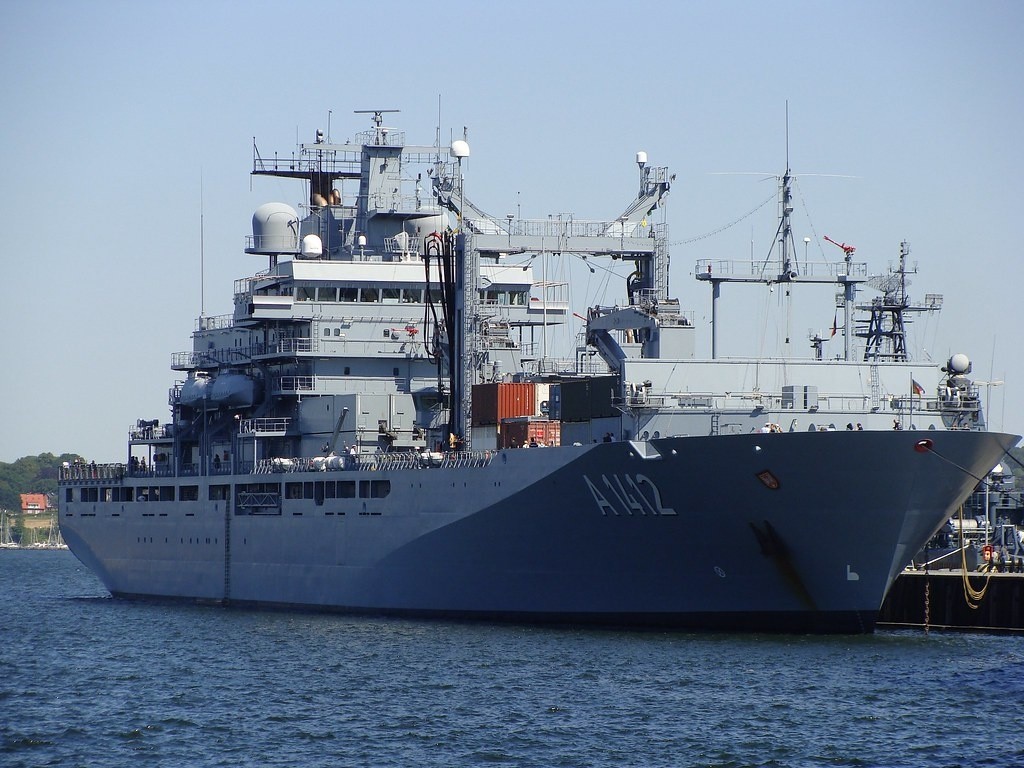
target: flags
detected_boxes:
[912,379,925,398]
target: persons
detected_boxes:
[509,433,616,449]
[846,423,863,430]
[344,445,356,471]
[997,545,1010,573]
[951,421,968,430]
[74,459,87,479]
[89,460,97,478]
[893,419,903,430]
[375,446,383,469]
[761,424,782,433]
[62,459,69,478]
[130,455,147,469]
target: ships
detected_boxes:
[58,89,1024,637]
[878,350,1024,632]
